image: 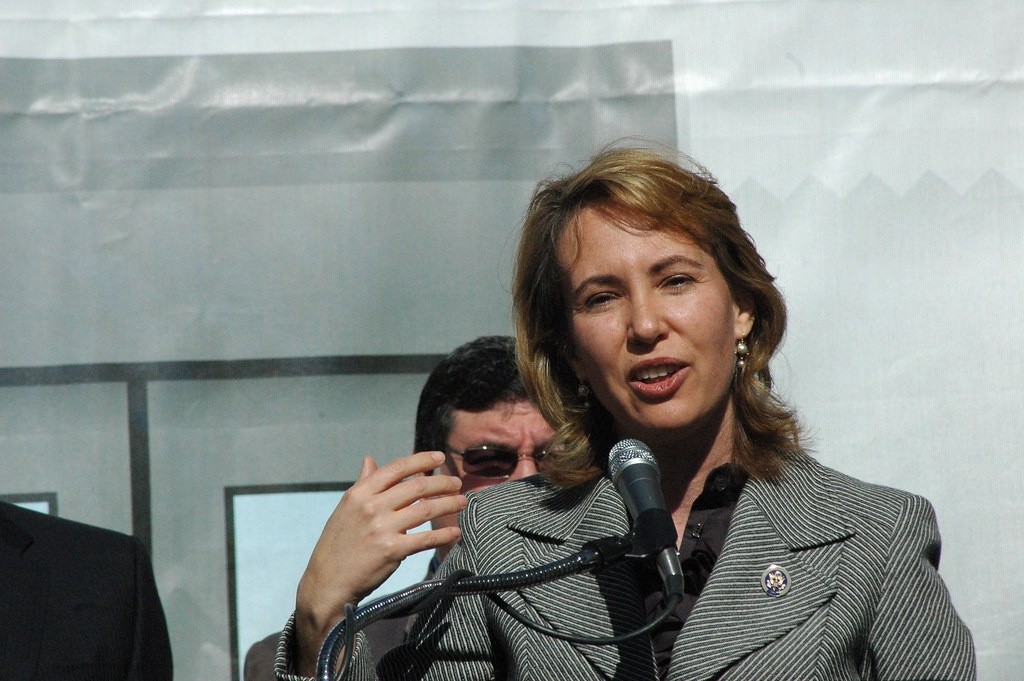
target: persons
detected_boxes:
[1,501,176,681]
[274,136,976,680]
[242,334,560,679]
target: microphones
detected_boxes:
[607,439,686,604]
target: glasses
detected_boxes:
[445,444,547,476]
